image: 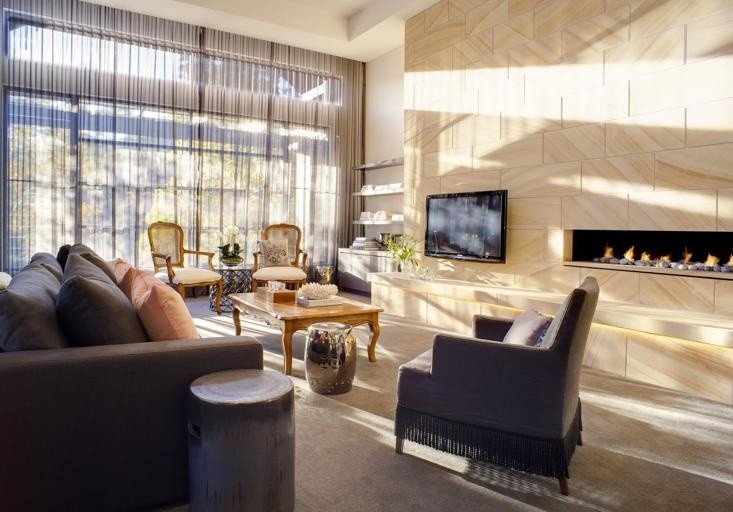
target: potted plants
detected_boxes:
[384,234,429,278]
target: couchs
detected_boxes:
[0,270,262,512]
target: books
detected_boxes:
[348,208,393,251]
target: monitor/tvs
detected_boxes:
[424,190,507,264]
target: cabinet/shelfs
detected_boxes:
[337,157,403,297]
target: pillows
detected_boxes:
[503,309,551,345]
[2,244,200,352]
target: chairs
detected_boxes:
[394,277,600,497]
[147,221,309,315]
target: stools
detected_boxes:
[303,322,357,394]
[316,264,335,284]
